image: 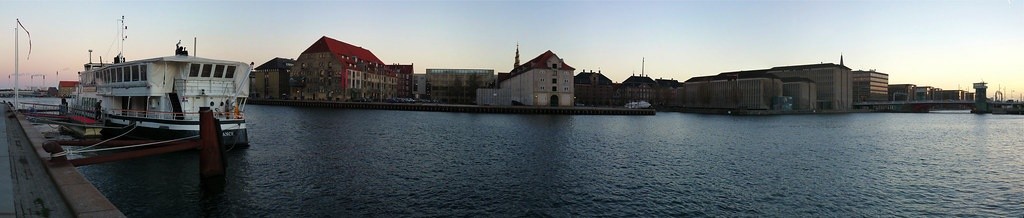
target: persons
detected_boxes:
[95,99,102,121]
[175,40,188,56]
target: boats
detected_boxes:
[69,15,255,147]
